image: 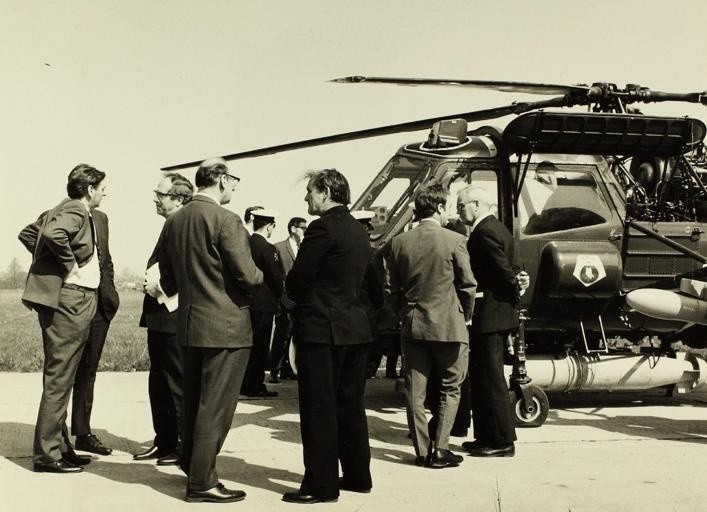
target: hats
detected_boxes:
[348,209,376,222]
[249,209,274,221]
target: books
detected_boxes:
[143,262,180,313]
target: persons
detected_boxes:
[16,166,107,474]
[238,206,307,399]
[157,157,266,501]
[131,171,195,467]
[66,163,119,456]
[347,182,529,468]
[280,165,378,505]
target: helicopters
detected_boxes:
[160,77,707,428]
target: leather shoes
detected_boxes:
[415,454,430,467]
[134,446,167,459]
[338,477,370,493]
[64,452,91,465]
[76,434,112,455]
[282,490,337,503]
[461,438,485,447]
[156,452,178,464]
[186,483,246,502]
[432,448,463,467]
[469,445,514,456]
[34,457,85,473]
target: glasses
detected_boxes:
[295,226,307,230]
[217,172,240,184]
[458,201,474,211]
[154,191,182,200]
